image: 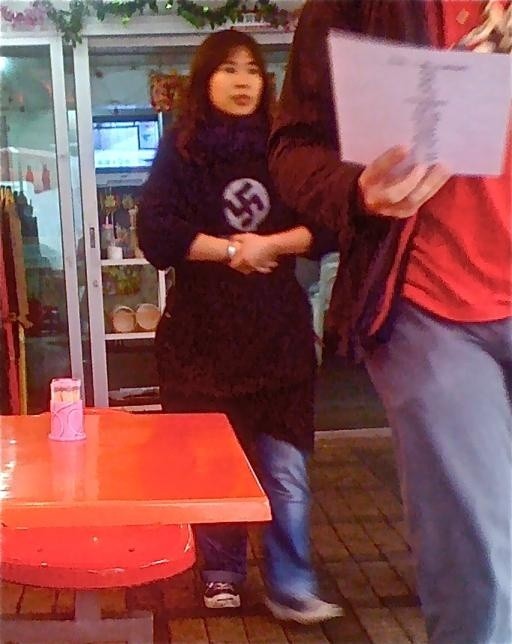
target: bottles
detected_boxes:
[26,166,33,189]
[43,162,50,189]
[100,188,138,258]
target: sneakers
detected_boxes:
[266,595,344,625]
[204,580,241,609]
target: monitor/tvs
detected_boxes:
[86,106,164,187]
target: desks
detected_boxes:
[0,412,272,530]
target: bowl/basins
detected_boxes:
[137,303,160,330]
[112,306,137,332]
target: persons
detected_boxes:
[265,0,512,644]
[136,30,345,625]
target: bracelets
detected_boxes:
[225,238,237,261]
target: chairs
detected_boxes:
[0,408,195,644]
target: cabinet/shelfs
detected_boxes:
[92,105,166,342]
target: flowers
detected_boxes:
[1,0,307,46]
